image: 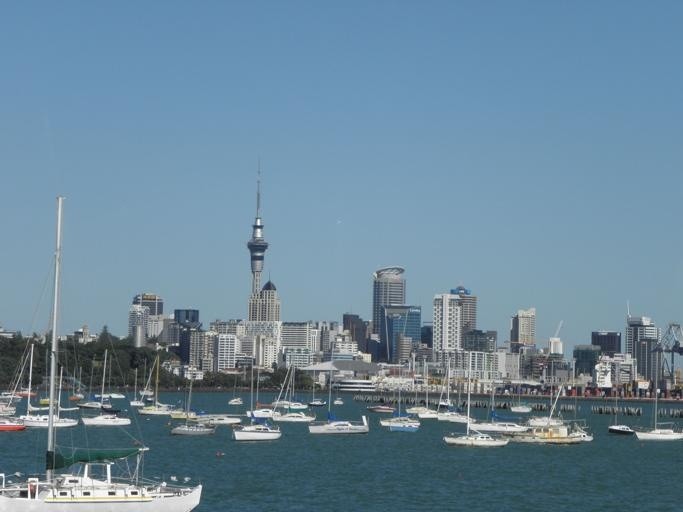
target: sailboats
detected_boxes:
[333,386,344,405]
[0,194,204,512]
[228,364,294,441]
[0,342,244,436]
[635,351,683,442]
[306,341,371,435]
[401,350,595,445]
[230,368,242,406]
[442,352,510,448]
[244,362,326,426]
[378,358,422,432]
[607,374,635,435]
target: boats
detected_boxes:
[366,405,396,414]
